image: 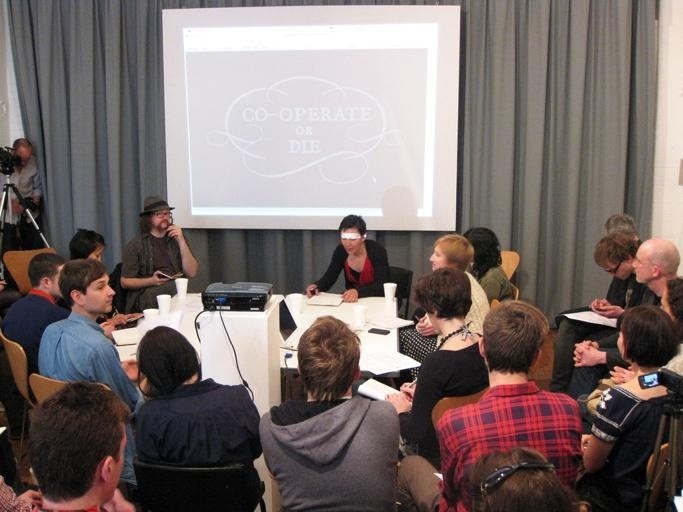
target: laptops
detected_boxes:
[278,296,304,350]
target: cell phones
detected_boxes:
[595,305,607,311]
[367,328,390,335]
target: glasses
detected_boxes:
[156,211,172,218]
[481,460,556,494]
[607,261,622,272]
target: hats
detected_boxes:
[139,194,175,216]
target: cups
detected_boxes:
[383,282,398,303]
[140,307,160,322]
[174,277,190,299]
[352,304,367,328]
[154,293,173,319]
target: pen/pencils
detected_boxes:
[114,306,119,314]
[408,378,417,388]
[311,286,319,292]
[415,315,423,324]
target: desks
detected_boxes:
[113,292,422,398]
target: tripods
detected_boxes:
[641,391,683,512]
[0,174,50,254]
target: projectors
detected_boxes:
[201,281,272,312]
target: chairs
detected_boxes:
[133,459,267,511]
[643,443,683,512]
[29,372,114,404]
[2,247,58,295]
[0,327,38,461]
[490,250,520,314]
[109,263,127,317]
[431,386,488,431]
[390,267,413,319]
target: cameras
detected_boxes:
[638,368,683,391]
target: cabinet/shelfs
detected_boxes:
[199,294,282,511]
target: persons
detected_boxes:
[260,316,400,512]
[469,447,575,512]
[134,325,263,512]
[553,213,683,401]
[581,303,679,499]
[399,234,490,381]
[397,300,584,512]
[69,228,105,260]
[462,228,513,302]
[38,259,146,487]
[2,252,67,376]
[27,381,132,512]
[307,214,403,309]
[120,195,199,313]
[0,138,42,288]
[386,267,490,461]
[0,475,42,512]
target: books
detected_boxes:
[358,379,400,401]
